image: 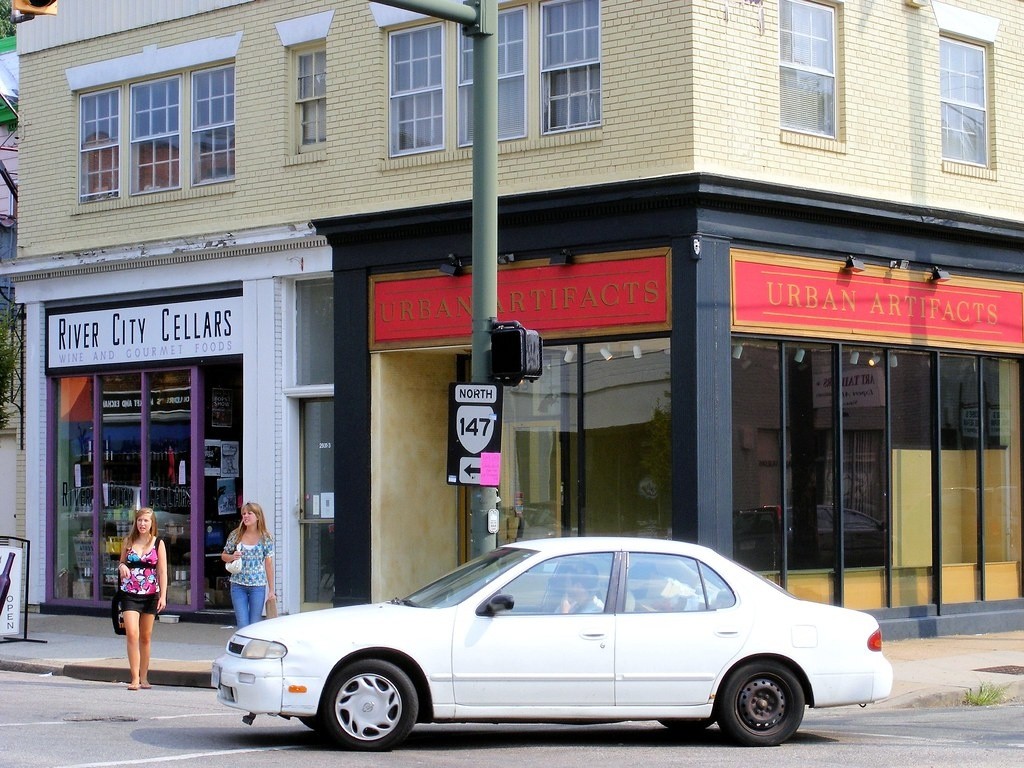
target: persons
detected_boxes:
[555,561,605,615]
[118,507,167,690]
[221,502,276,630]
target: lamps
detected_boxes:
[498,253,515,265]
[732,345,743,359]
[564,347,574,363]
[927,266,952,283]
[890,352,898,368]
[632,341,642,359]
[869,352,880,366]
[844,254,866,273]
[794,348,805,363]
[438,252,465,277]
[549,248,573,265]
[889,259,910,270]
[599,347,613,362]
[849,350,859,364]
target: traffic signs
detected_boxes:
[444,381,505,489]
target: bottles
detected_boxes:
[174,565,180,580]
[105,560,120,584]
[0,552,15,615]
[181,565,187,580]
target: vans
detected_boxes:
[60,478,194,557]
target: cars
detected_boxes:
[210,535,892,754]
[732,503,888,571]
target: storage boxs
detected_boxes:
[300,602,334,614]
[72,581,93,600]
[167,578,227,605]
[157,615,180,624]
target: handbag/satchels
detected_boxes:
[224,542,242,574]
[112,562,127,635]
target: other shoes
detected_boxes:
[128,681,139,690]
[141,680,151,689]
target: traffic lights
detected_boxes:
[490,327,541,378]
[13,0,60,16]
